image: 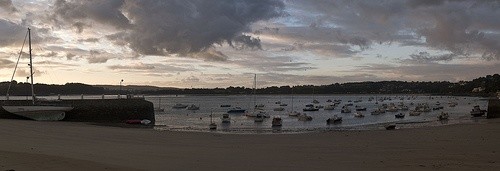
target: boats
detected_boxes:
[384,125,397,130]
[186,104,201,110]
[220,104,231,107]
[253,113,264,122]
[273,95,471,124]
[220,113,231,123]
[172,103,189,109]
[227,106,245,113]
[125,119,151,124]
[470,105,487,117]
[271,117,284,126]
[257,103,265,107]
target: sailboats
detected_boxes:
[209,109,217,129]
[1,28,75,121]
[245,74,270,118]
